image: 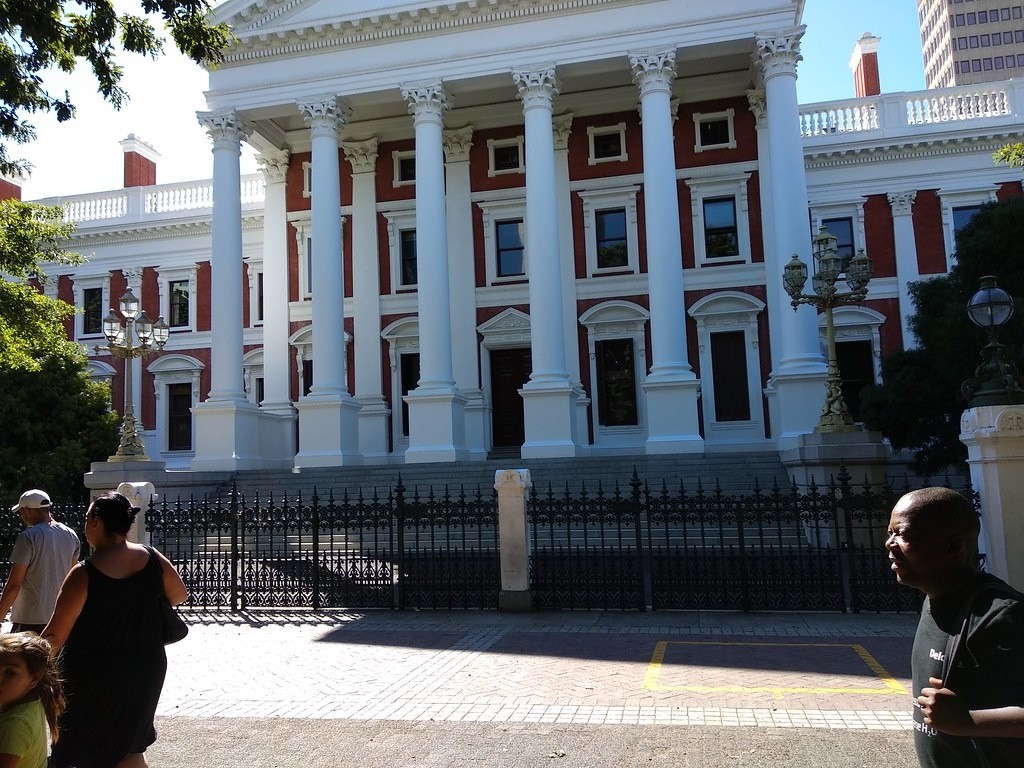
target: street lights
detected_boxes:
[94,287,170,462]
[781,225,876,433]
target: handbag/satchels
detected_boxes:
[144,546,188,645]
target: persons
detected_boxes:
[0,489,81,635]
[0,631,73,768]
[41,490,187,768]
[885,487,1024,768]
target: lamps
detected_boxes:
[960,275,1024,408]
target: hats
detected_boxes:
[12,489,52,514]
